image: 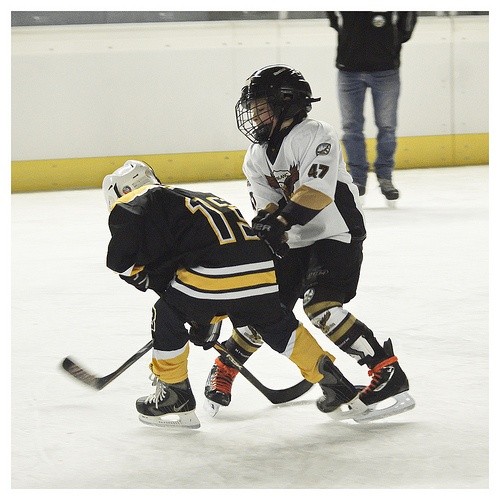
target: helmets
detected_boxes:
[235,64,321,145]
[101,160,161,213]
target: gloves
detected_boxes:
[252,208,291,238]
[148,267,174,291]
[189,321,222,350]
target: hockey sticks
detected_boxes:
[156,290,313,404]
[62,339,153,391]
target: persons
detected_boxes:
[327,11,420,200]
[102,160,358,414]
[204,64,409,405]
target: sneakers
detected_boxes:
[348,337,416,422]
[378,178,399,207]
[317,354,376,421]
[204,341,239,418]
[352,182,365,196]
[136,374,201,429]
